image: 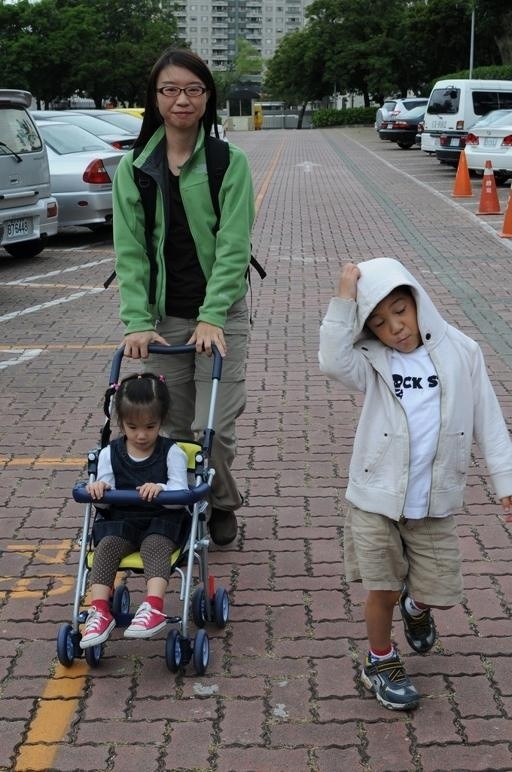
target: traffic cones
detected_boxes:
[472,158,506,218]
[496,182,512,239]
[447,148,476,198]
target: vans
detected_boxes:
[416,77,512,178]
[0,85,60,261]
[258,102,285,116]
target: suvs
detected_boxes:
[374,94,432,151]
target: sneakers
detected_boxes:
[398,590,437,655]
[79,605,117,650]
[359,646,420,712]
[123,600,168,641]
[208,505,239,546]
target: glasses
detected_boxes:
[154,85,208,99]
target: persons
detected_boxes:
[76,372,189,648]
[316,256,512,708]
[111,47,256,545]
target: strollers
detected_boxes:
[54,337,231,681]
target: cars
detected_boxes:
[463,106,512,186]
[32,104,229,239]
[378,103,428,141]
